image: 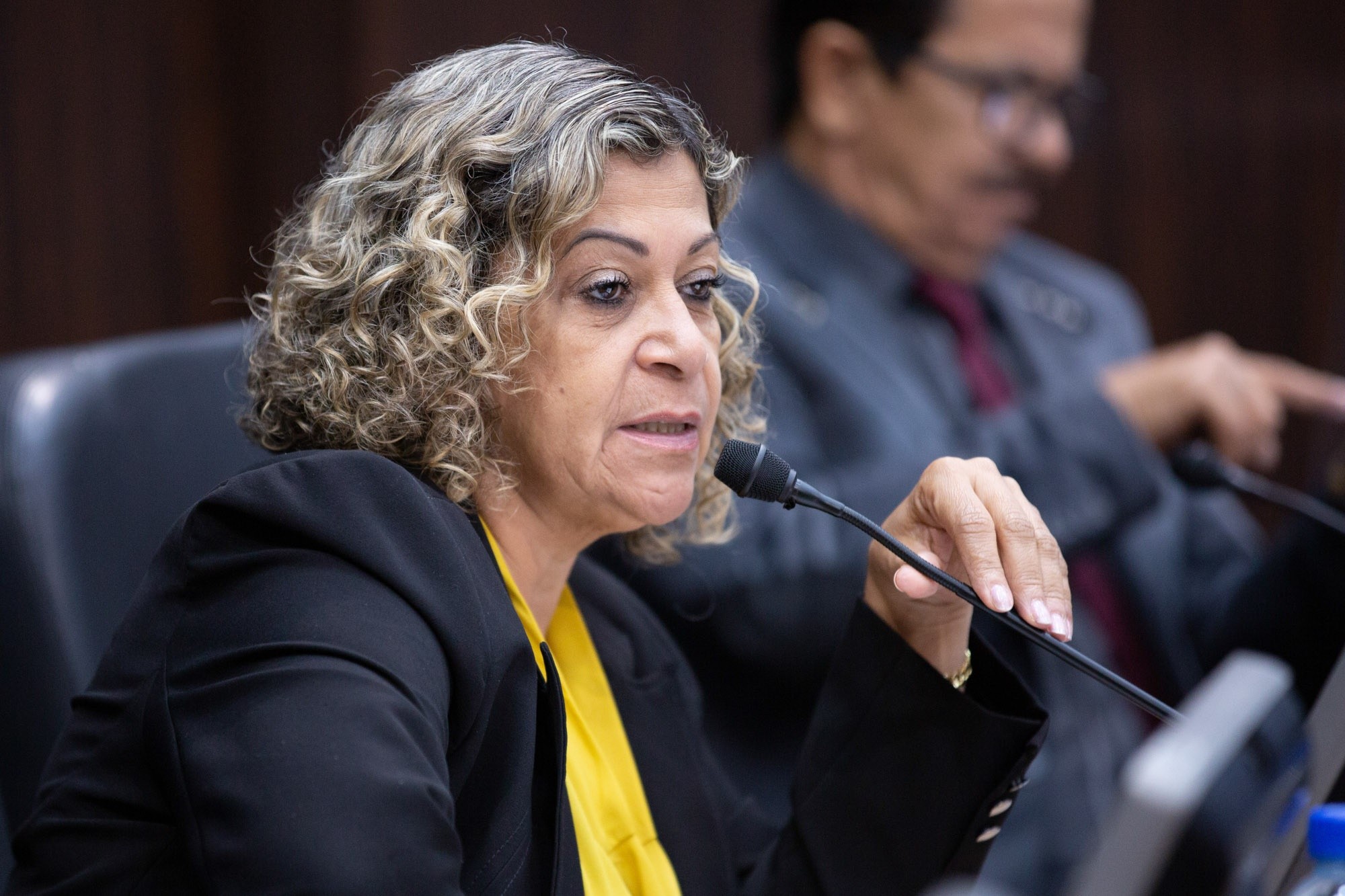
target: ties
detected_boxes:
[909,271,1161,738]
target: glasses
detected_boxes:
[912,43,1093,156]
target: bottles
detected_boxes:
[1284,803,1345,896]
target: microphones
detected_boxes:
[1172,439,1345,537]
[715,437,1301,895]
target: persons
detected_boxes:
[583,0,1345,896]
[0,42,1076,896]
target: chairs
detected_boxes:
[0,320,292,841]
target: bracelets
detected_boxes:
[940,648,974,691]
